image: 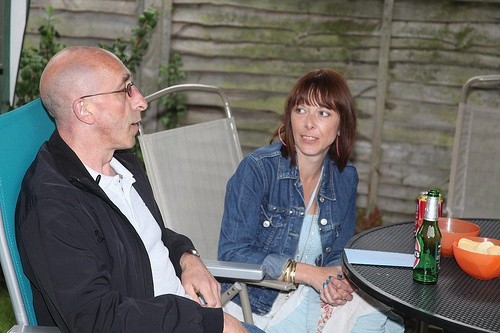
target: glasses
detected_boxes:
[81,83,133,98]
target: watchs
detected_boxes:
[186,249,200,257]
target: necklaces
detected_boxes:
[285,189,320,301]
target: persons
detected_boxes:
[14,46,267,333]
[216,69,405,333]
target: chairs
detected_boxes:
[0,98,264,332]
[445,73,500,221]
[138,84,296,325]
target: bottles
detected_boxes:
[413,190,442,284]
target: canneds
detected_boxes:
[413,192,443,237]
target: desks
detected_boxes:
[341,219,500,332]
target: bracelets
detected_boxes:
[282,260,299,289]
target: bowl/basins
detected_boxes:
[453,237,500,280]
[418,218,480,257]
